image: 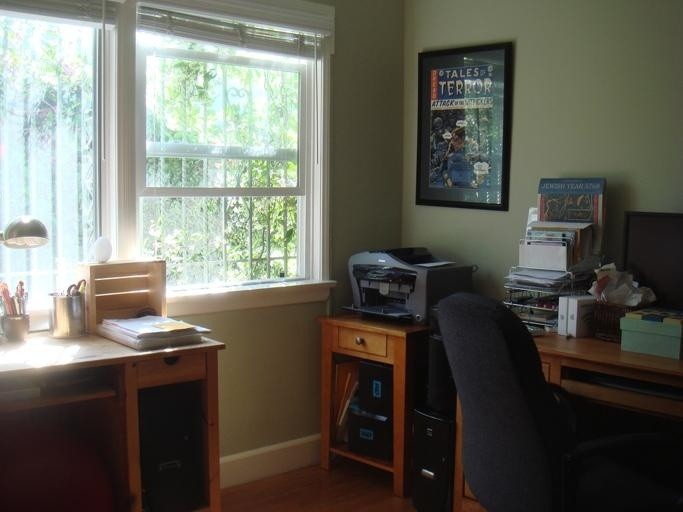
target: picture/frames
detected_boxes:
[415,42,512,211]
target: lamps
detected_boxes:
[1,219,48,249]
[92,236,111,263]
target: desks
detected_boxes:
[452,331,683,511]
[315,313,428,497]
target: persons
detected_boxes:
[429,116,489,189]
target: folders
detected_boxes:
[93,313,212,351]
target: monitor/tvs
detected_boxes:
[614,210,682,310]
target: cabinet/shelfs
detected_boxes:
[0,330,226,511]
[505,266,598,331]
[435,348,682,510]
[79,258,166,334]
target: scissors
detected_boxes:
[67,280,86,296]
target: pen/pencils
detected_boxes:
[0,281,28,316]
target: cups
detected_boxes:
[1,314,29,342]
[47,295,86,338]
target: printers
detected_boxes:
[338,244,473,327]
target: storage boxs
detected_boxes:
[620,317,683,360]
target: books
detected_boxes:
[504,177,607,337]
[94,315,213,352]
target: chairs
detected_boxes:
[436,291,683,512]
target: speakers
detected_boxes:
[426,302,458,420]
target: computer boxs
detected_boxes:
[408,407,456,511]
